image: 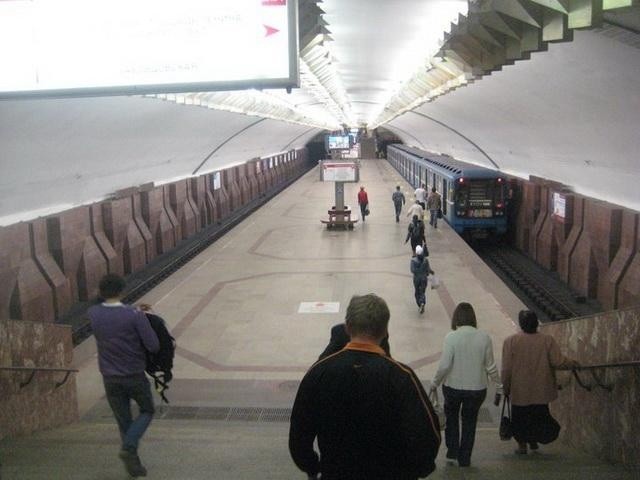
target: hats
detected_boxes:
[415,244,424,257]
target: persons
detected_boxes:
[287,294,442,478]
[87,273,160,476]
[319,323,391,358]
[500,308,578,454]
[355,186,373,221]
[429,302,503,467]
[392,183,440,310]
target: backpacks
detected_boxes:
[142,306,175,382]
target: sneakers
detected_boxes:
[417,303,425,314]
[429,222,437,229]
[119,450,146,477]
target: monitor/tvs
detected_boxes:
[324,135,353,151]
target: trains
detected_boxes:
[386,144,510,247]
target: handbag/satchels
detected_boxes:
[428,388,448,431]
[536,412,562,445]
[423,244,429,257]
[499,395,512,441]
[365,209,370,216]
[437,208,443,219]
